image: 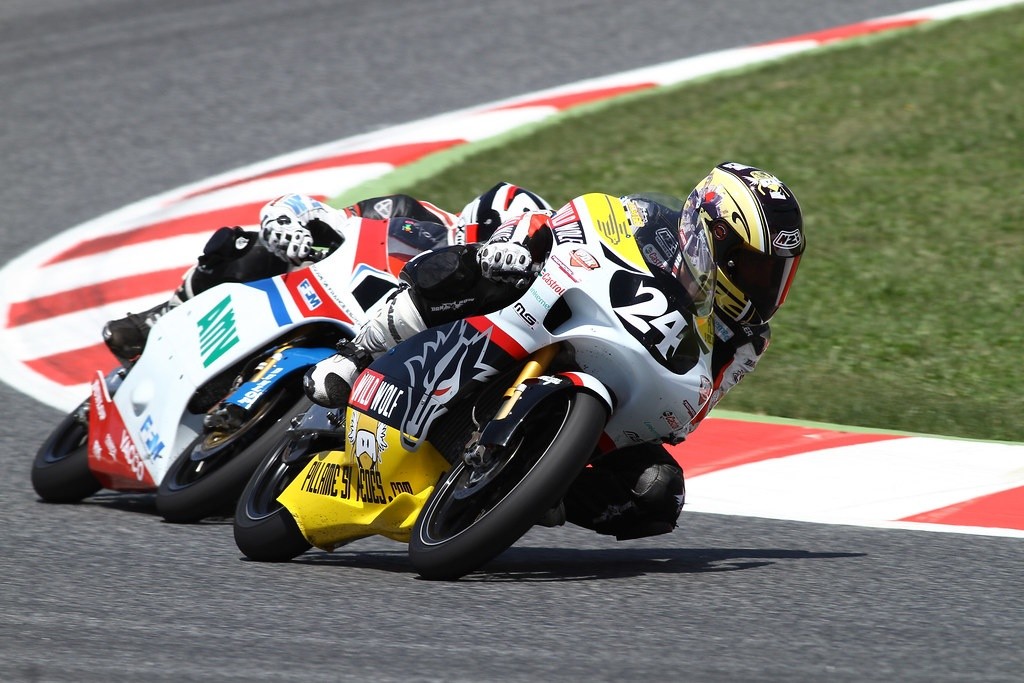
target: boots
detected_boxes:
[102,263,199,360]
[302,283,428,410]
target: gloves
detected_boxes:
[258,214,314,261]
[476,239,533,290]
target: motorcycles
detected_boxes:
[233,194,718,580]
[31,211,457,524]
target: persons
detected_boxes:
[100,179,552,357]
[302,164,807,540]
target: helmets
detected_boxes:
[458,181,556,228]
[677,160,807,326]
